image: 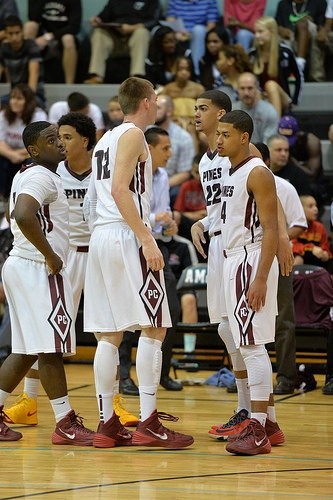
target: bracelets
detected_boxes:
[45,33,53,41]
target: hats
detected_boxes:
[278,116,298,146]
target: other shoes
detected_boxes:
[84,78,101,84]
[323,379,333,394]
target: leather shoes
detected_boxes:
[274,382,294,393]
[227,383,238,392]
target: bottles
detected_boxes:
[161,206,172,235]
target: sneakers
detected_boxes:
[226,424,271,455]
[207,410,252,440]
[51,409,96,445]
[0,393,37,425]
[0,405,23,440]
[160,377,183,390]
[132,410,194,448]
[95,410,134,447]
[227,418,284,445]
[119,378,140,395]
[112,393,139,427]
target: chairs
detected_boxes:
[171,262,329,386]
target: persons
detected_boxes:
[0,0,333,454]
